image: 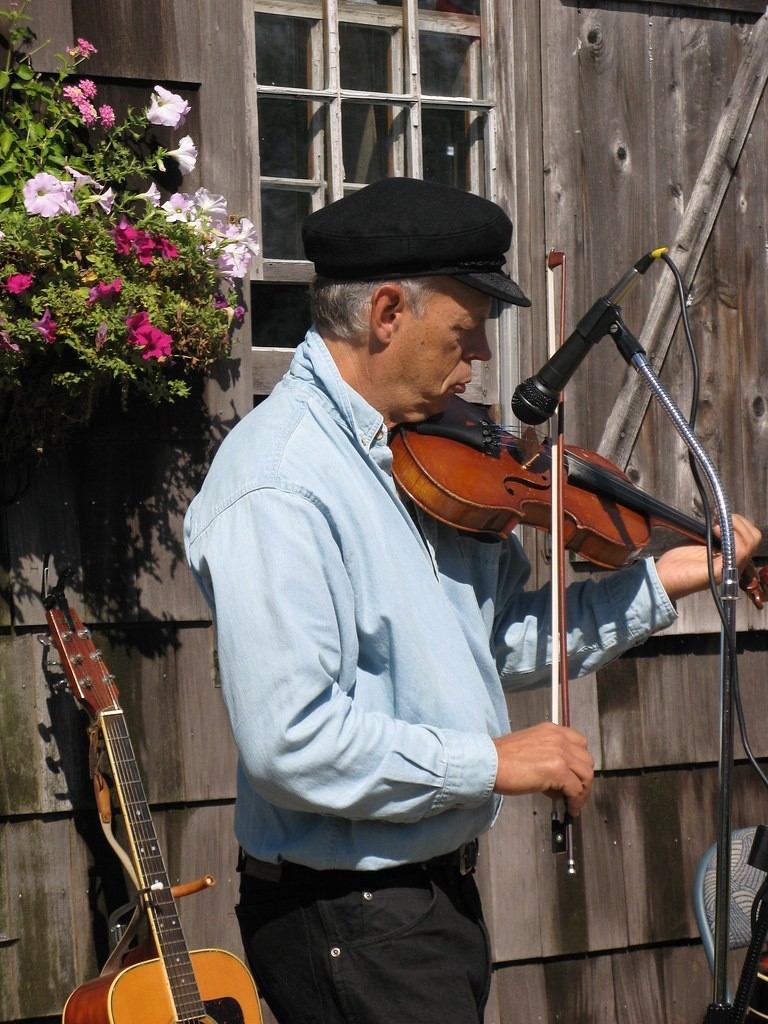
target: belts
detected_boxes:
[243,840,478,886]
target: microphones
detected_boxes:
[511,252,656,426]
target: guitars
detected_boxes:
[30,561,264,1024]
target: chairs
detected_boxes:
[693,826,767,1009]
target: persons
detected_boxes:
[175,176,761,1024]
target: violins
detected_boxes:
[389,391,768,614]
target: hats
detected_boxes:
[301,177,533,307]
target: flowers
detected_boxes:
[0,0,262,465]
